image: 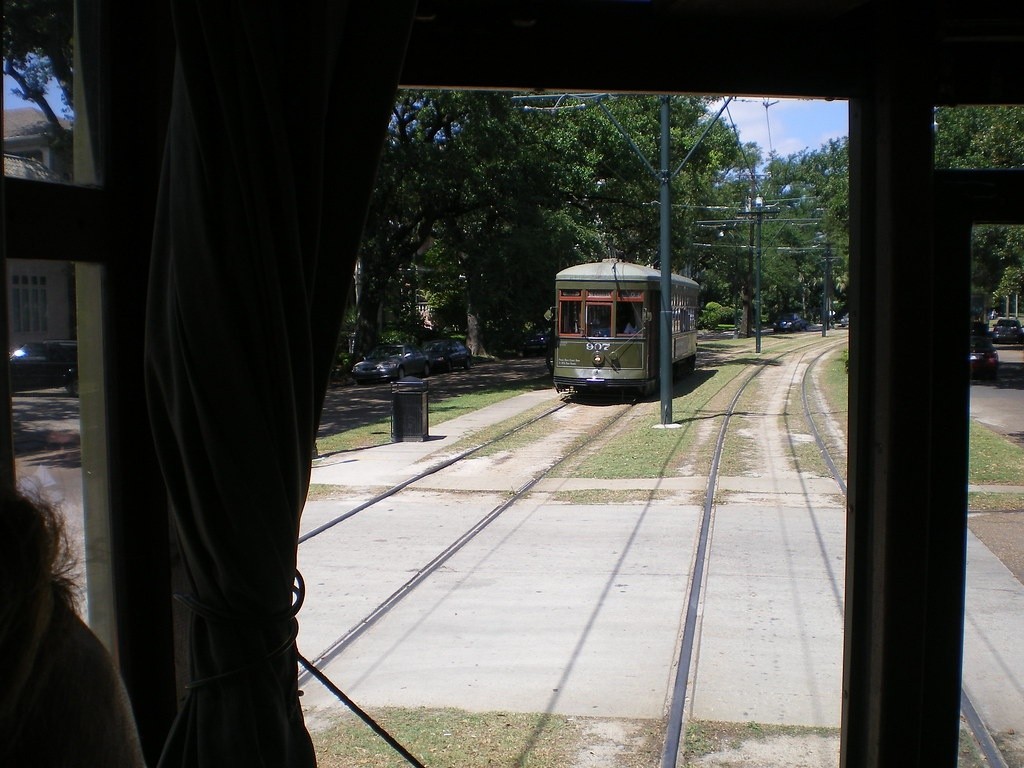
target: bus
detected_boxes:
[544,258,699,398]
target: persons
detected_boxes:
[624,316,637,333]
[831,318,836,329]
[991,309,998,319]
[593,316,611,337]
[0,484,147,768]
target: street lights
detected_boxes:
[756,235,827,353]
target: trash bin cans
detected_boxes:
[388,380,430,443]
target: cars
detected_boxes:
[9,341,78,397]
[773,313,805,332]
[841,313,849,327]
[351,343,429,384]
[970,322,999,381]
[992,319,1024,344]
[423,340,473,373]
